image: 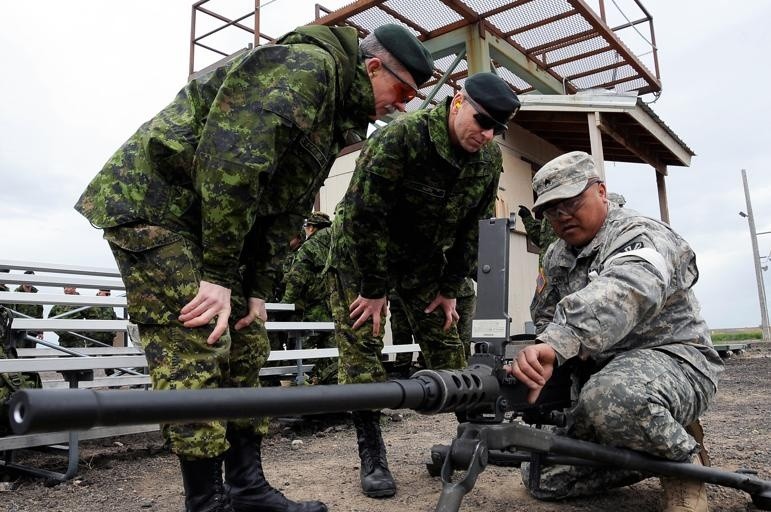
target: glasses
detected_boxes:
[540,180,606,222]
[362,54,418,105]
[464,96,509,136]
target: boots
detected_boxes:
[662,452,711,512]
[348,409,397,498]
[177,454,226,512]
[686,419,712,466]
[225,421,328,512]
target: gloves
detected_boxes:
[518,205,531,219]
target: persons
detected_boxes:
[73,24,436,511]
[389,287,426,375]
[281,211,339,386]
[280,227,306,300]
[502,149,726,512]
[0,266,118,405]
[454,277,474,361]
[322,73,521,501]
[518,206,559,269]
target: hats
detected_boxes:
[532,151,602,212]
[465,73,522,125]
[375,24,435,90]
[307,211,333,225]
